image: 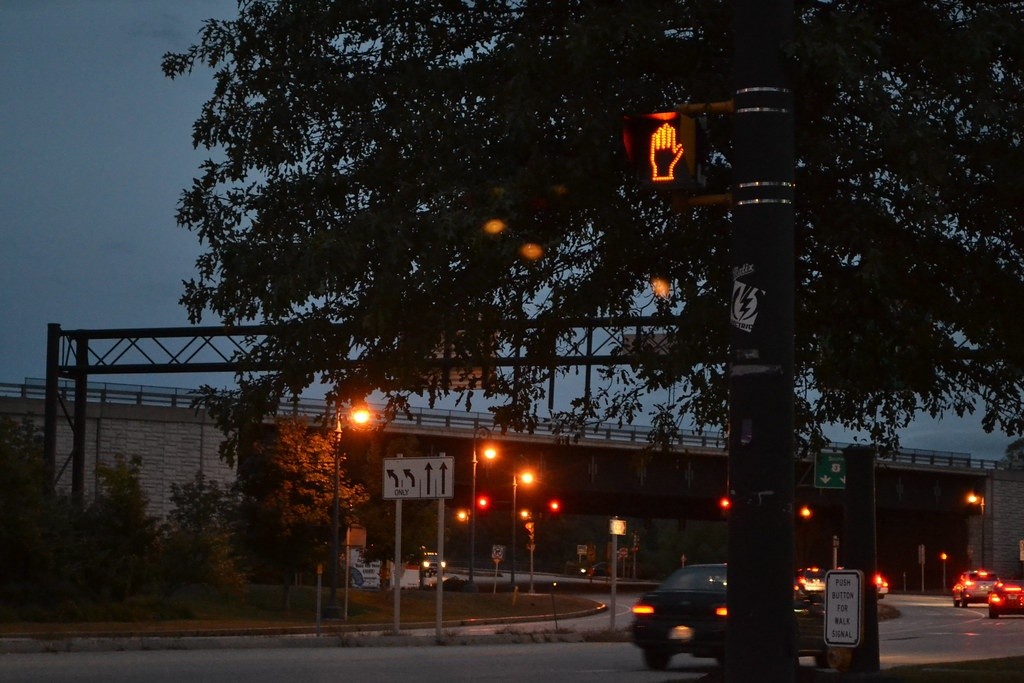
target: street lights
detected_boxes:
[465,424,496,591]
[327,384,374,620]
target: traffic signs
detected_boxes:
[382,455,453,501]
[823,568,865,648]
[812,449,847,490]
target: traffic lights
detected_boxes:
[623,107,694,191]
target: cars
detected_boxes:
[951,570,1001,608]
[629,565,846,671]
[418,552,447,575]
[987,579,1023,619]
